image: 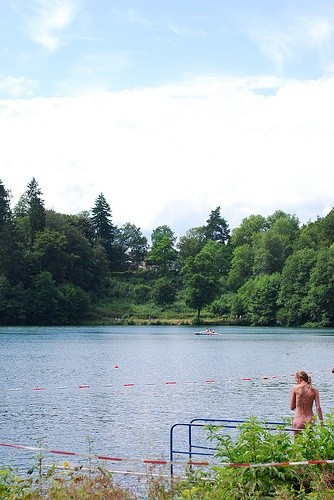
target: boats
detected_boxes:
[194,330,218,334]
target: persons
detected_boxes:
[204,327,215,333]
[289,370,324,444]
